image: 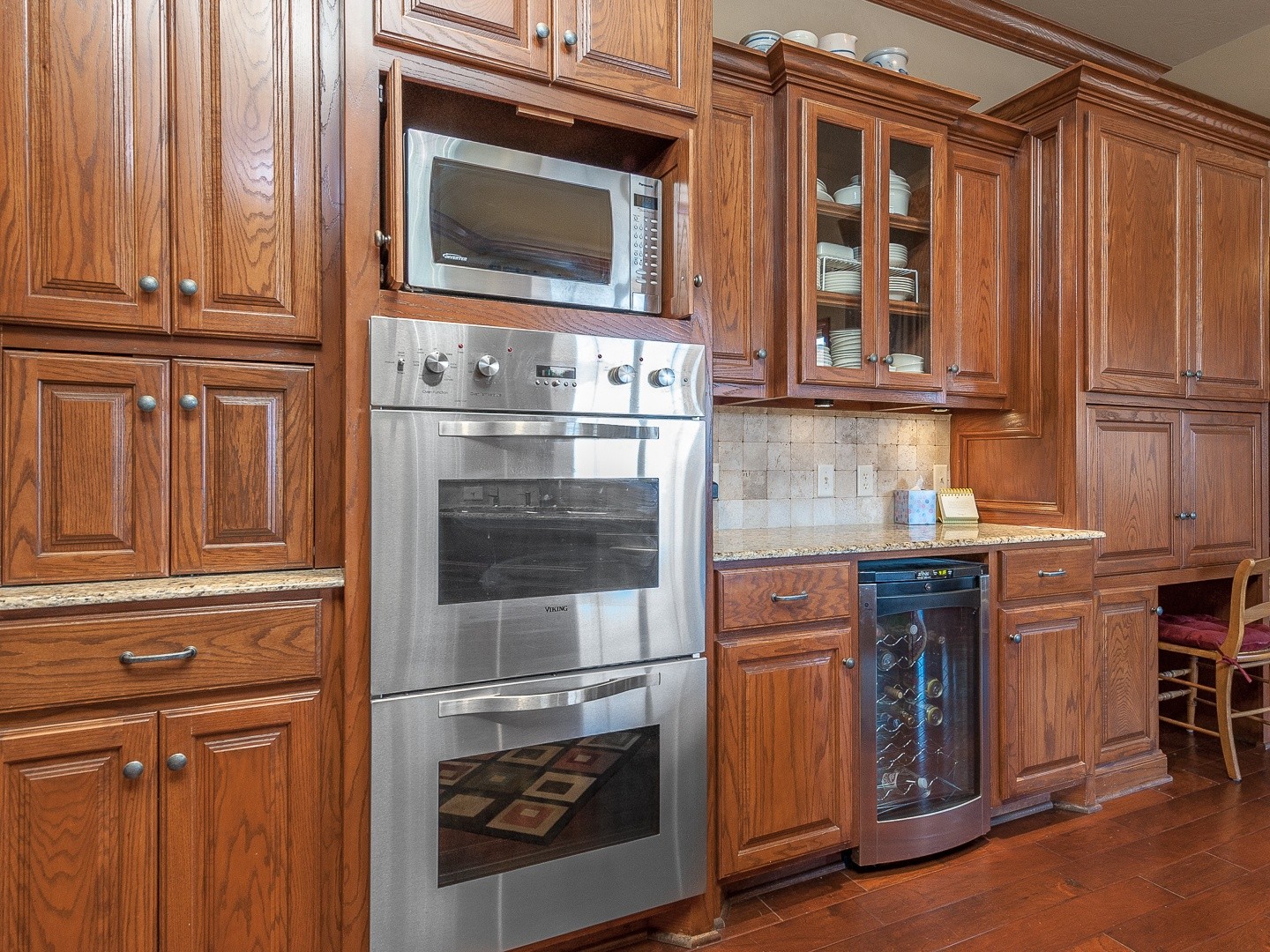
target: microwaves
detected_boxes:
[404,127,666,317]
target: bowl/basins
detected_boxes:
[739,29,784,54]
[833,166,912,217]
[783,30,819,48]
[889,353,925,373]
[862,46,910,76]
[819,32,858,59]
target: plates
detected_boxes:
[816,326,861,369]
[817,242,915,300]
[817,178,835,202]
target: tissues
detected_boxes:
[894,475,937,525]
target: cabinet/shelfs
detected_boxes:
[0,0,1270,952]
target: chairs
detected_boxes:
[1158,557,1270,781]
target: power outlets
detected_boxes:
[857,465,873,497]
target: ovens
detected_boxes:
[364,316,709,951]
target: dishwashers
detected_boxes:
[854,554,999,869]
